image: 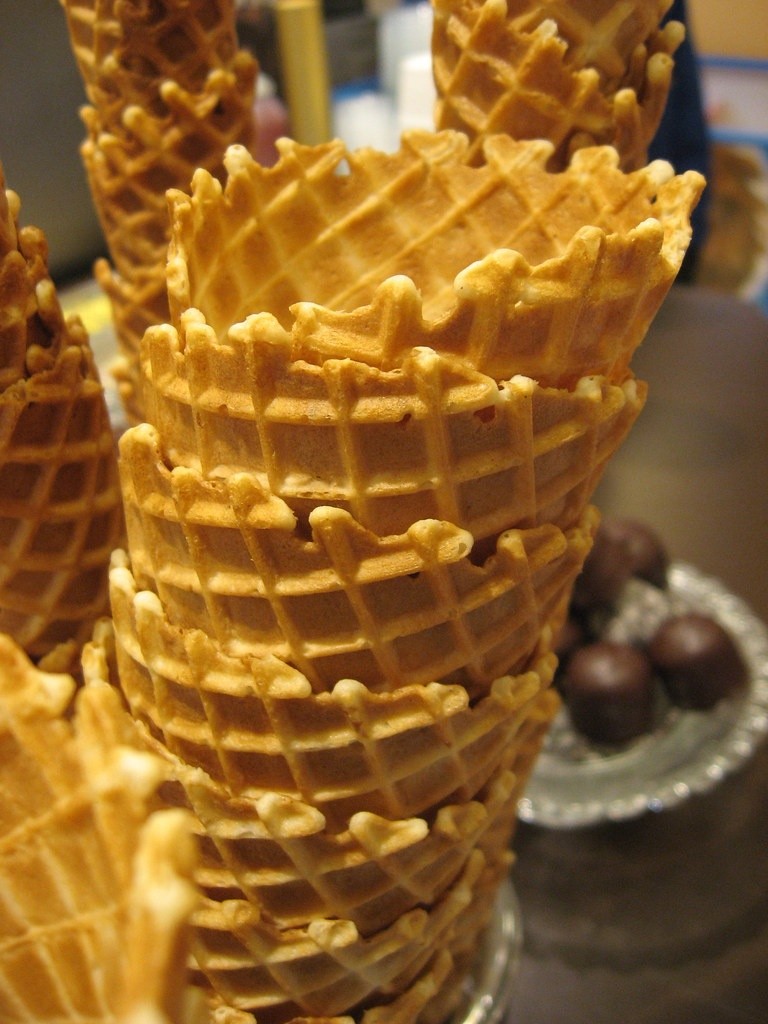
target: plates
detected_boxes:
[455,877,522,1024]
[511,562,768,827]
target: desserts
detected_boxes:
[557,516,742,749]
